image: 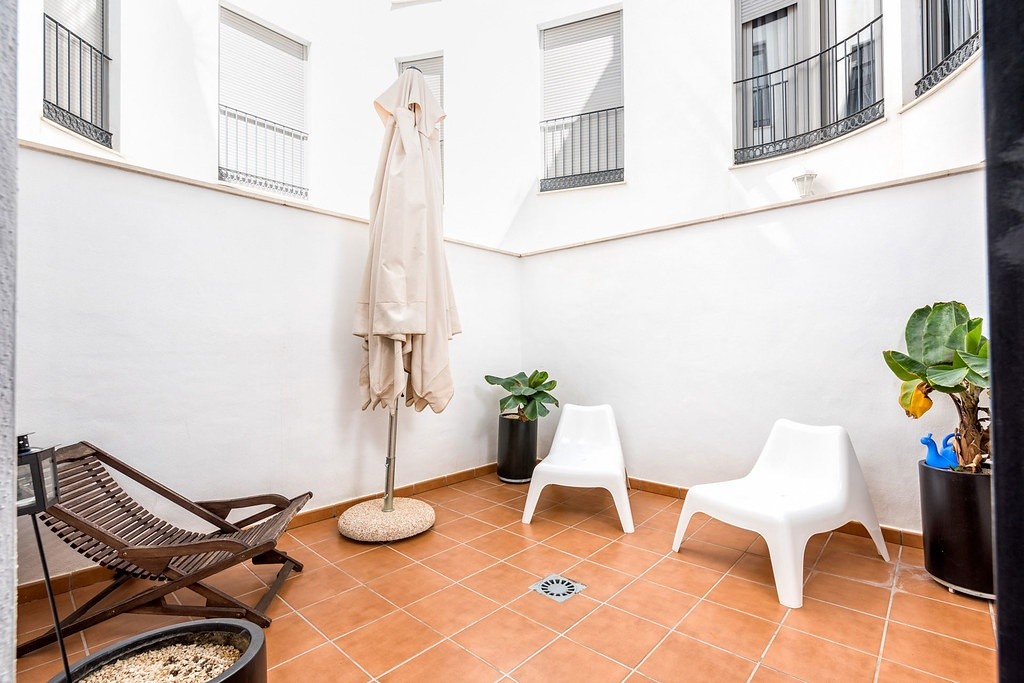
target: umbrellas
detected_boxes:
[353,69,459,514]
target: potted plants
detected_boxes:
[883,300,996,602]
[485,369,560,484]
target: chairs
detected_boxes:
[670,418,890,609]
[15,441,314,656]
[520,404,639,534]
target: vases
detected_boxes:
[51,617,267,683]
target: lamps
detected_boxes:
[17,432,75,683]
